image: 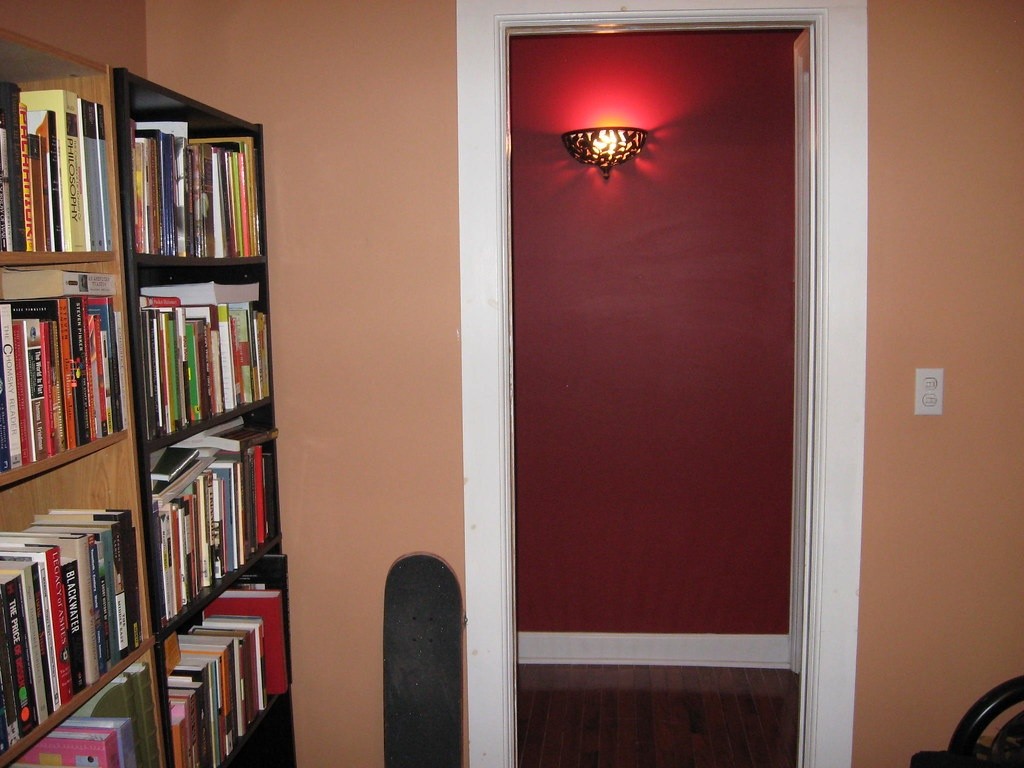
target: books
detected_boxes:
[1,267,123,475]
[149,416,276,621]
[164,555,289,768]
[0,79,112,251]
[0,507,143,755]
[7,662,161,768]
[138,282,270,436]
[132,118,262,259]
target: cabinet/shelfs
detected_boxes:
[0,27,298,768]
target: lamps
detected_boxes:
[561,127,648,179]
